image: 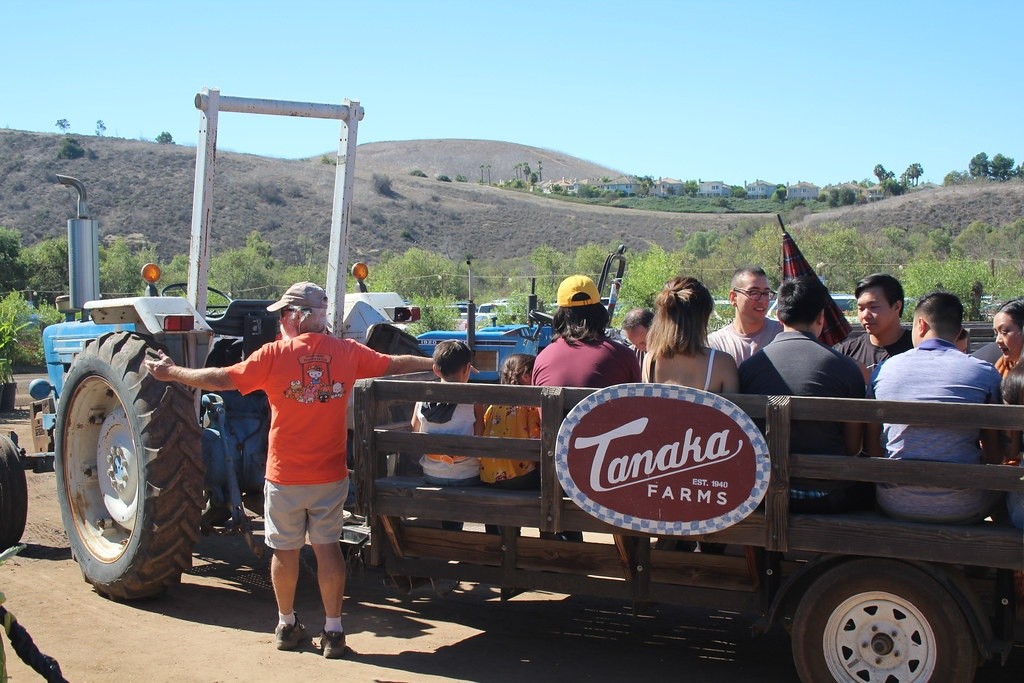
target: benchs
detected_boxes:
[369,475,1024,573]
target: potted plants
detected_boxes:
[0,307,35,412]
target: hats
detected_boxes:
[266,281,327,312]
[557,275,600,307]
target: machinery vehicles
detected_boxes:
[10,86,1024,683]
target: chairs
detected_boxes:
[205,297,280,338]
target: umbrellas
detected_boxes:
[777,214,854,348]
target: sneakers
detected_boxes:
[275,609,307,651]
[319,627,346,659]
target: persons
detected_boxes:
[409,268,1024,605]
[143,281,481,657]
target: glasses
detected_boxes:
[732,287,775,301]
[280,307,311,320]
